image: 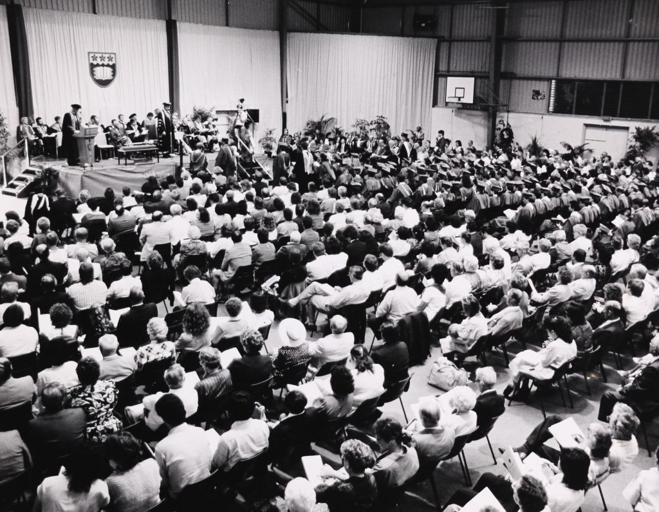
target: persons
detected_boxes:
[2,96,659,512]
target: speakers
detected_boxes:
[412,14,438,31]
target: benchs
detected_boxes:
[118,148,159,165]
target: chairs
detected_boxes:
[16,125,122,162]
[0,174,659,512]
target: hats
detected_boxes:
[278,318,307,348]
